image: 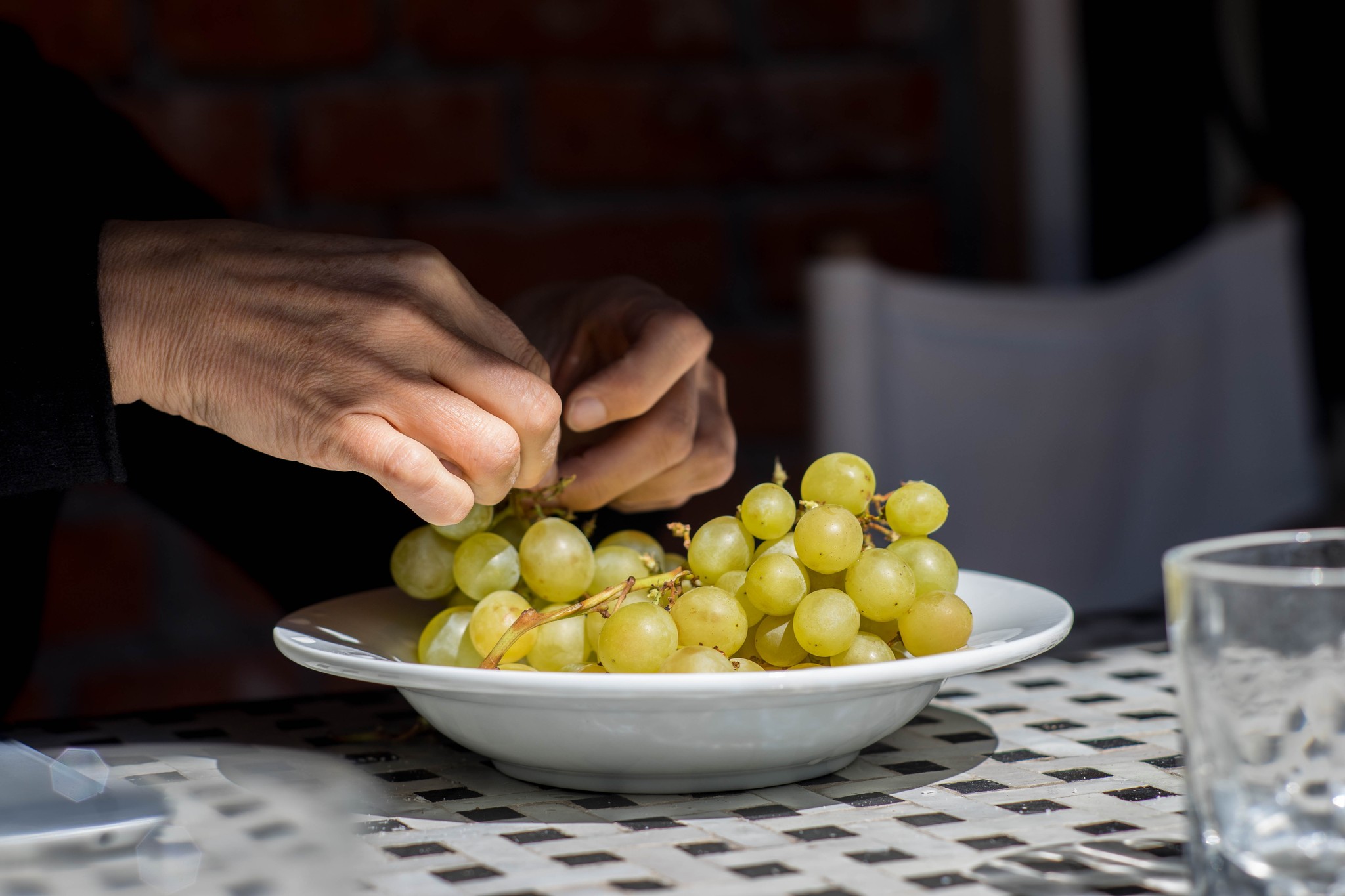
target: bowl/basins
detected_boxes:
[272,568,1074,794]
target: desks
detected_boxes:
[0,607,1345,895]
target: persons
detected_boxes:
[0,16,736,735]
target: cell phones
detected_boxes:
[0,737,170,861]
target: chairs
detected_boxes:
[802,198,1327,612]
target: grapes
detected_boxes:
[391,451,973,677]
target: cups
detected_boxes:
[1162,526,1345,895]
